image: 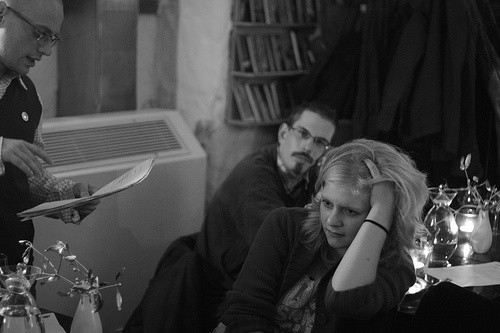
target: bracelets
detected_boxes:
[363,220,389,235]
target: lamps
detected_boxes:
[455,204,487,269]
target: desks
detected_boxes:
[399,259,500,316]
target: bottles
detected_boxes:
[0,264,44,333]
[426,186,459,268]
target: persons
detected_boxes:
[196,102,339,292]
[212,138,429,333]
[0,0,100,305]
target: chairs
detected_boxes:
[407,283,500,333]
[127,231,201,333]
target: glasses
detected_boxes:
[7,6,62,49]
[290,125,331,153]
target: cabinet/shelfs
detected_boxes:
[231,0,321,132]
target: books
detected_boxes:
[232,0,322,121]
